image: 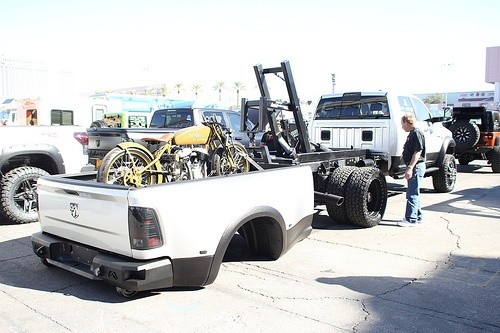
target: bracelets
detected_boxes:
[407,165,413,170]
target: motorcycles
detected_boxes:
[94,111,250,186]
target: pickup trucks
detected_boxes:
[86,106,266,170]
[30,59,458,298]
[0,96,99,223]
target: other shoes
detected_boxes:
[397,216,423,226]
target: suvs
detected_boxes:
[442,105,500,174]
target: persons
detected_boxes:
[397,114,426,226]
